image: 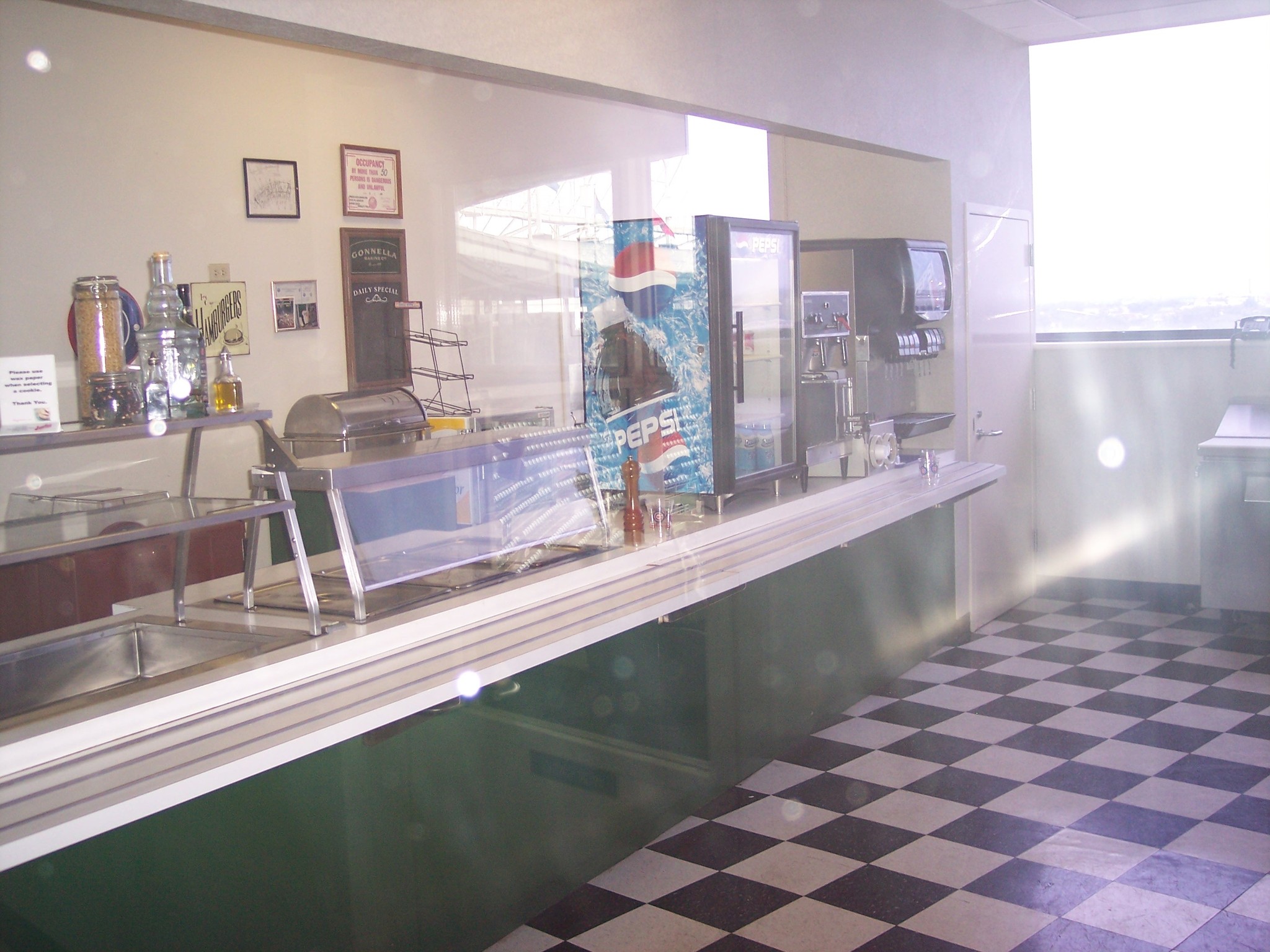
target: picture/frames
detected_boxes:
[243,158,300,218]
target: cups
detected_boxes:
[918,450,941,487]
[646,499,675,537]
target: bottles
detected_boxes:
[178,284,208,396]
[592,298,701,495]
[73,275,123,425]
[135,251,206,420]
[212,346,244,415]
[88,373,140,429]
[143,351,172,423]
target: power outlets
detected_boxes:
[209,264,231,282]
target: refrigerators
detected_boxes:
[580,215,809,515]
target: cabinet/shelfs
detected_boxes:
[393,301,481,417]
[0,410,322,636]
